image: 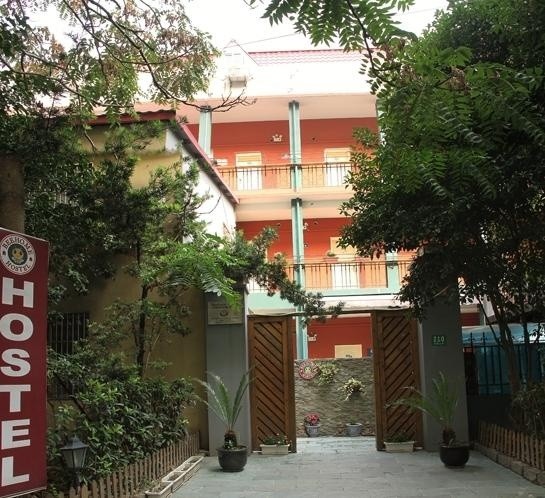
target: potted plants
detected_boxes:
[346,416,362,437]
[383,434,415,452]
[191,363,259,472]
[385,373,471,468]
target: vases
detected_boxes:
[260,444,292,455]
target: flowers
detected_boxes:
[306,425,320,437]
[303,414,320,426]
[260,434,290,449]
